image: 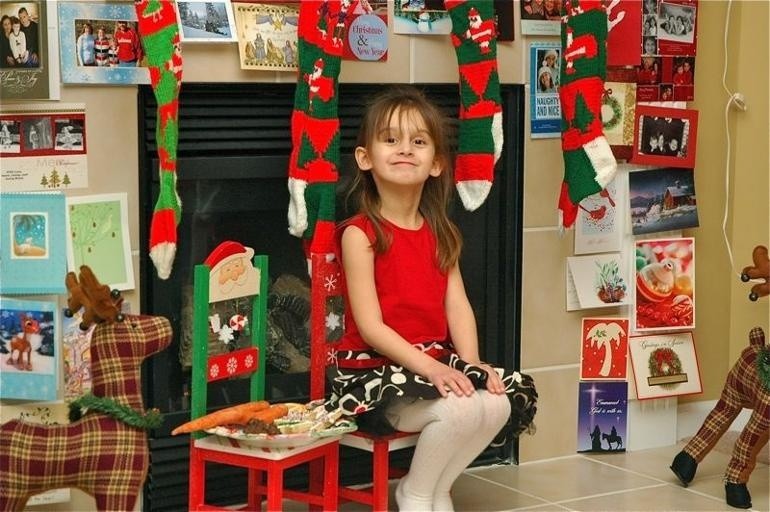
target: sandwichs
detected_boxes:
[270,404,347,435]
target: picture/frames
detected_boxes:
[57,2,152,85]
[630,105,699,169]
[0,1,51,100]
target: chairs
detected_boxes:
[188,241,343,511]
[308,253,452,512]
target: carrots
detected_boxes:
[172,398,288,436]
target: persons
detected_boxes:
[590,424,601,450]
[76,20,144,68]
[0,7,39,66]
[522,0,562,21]
[0,123,12,149]
[543,50,559,84]
[331,89,538,510]
[537,65,557,94]
[29,125,40,150]
[245,32,298,64]
[60,126,74,149]
[610,426,617,441]
[643,0,692,157]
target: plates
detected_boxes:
[205,420,359,447]
